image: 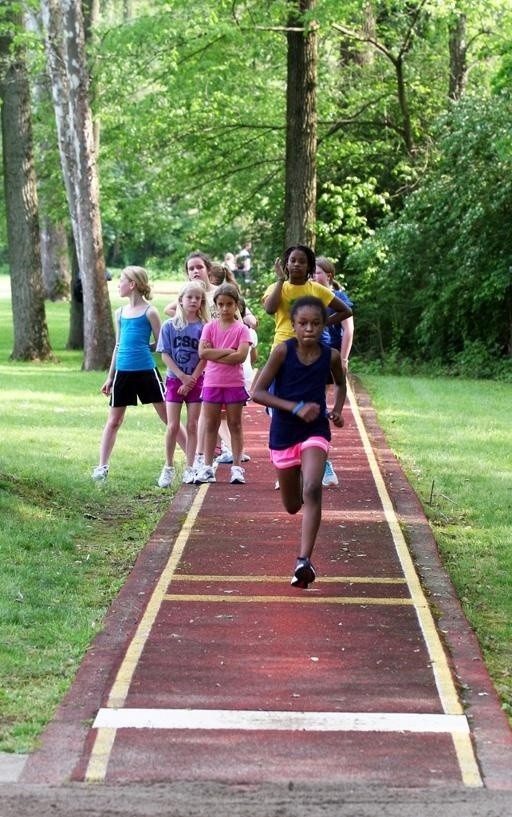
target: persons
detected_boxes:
[92,266,187,480]
[157,242,259,487]
[309,257,356,404]
[248,296,347,588]
[261,245,352,490]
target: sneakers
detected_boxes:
[291,559,316,589]
[158,464,175,487]
[183,453,246,484]
[92,466,109,481]
[322,460,338,486]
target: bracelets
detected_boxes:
[278,277,286,282]
[292,400,304,415]
